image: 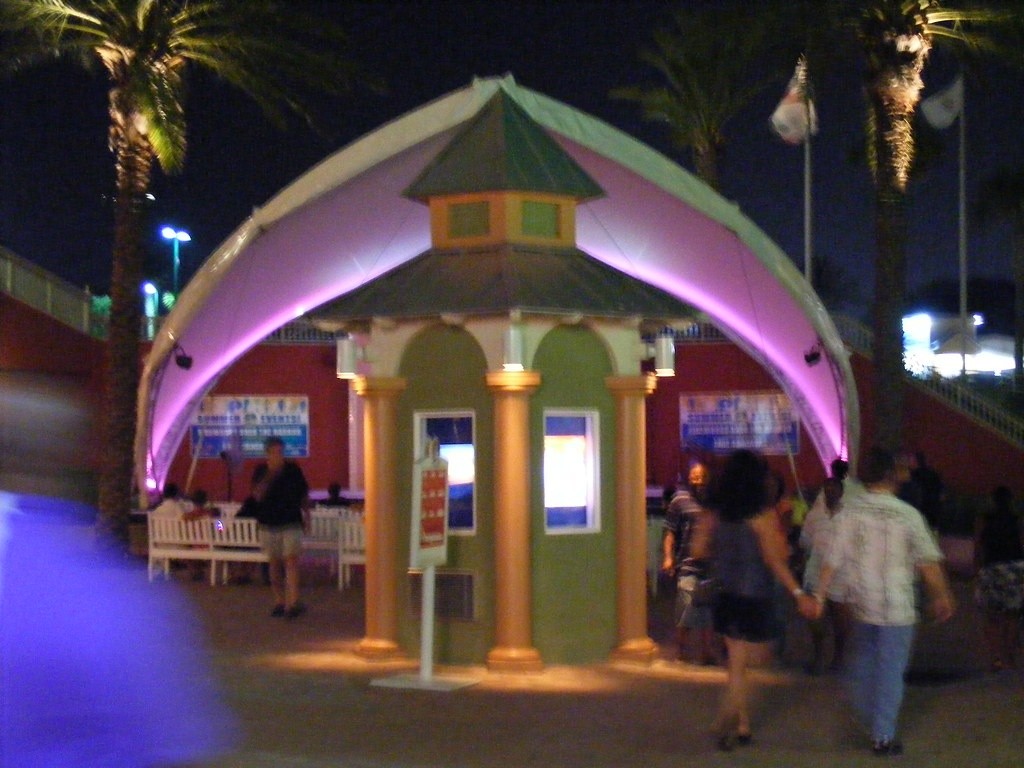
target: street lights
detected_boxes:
[772,53,821,287]
[159,226,193,300]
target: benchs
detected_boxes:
[146,499,365,592]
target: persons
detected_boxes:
[250,437,310,617]
[156,484,217,580]
[663,446,1024,757]
[0,489,230,768]
[236,497,285,586]
[220,434,244,499]
[319,482,352,507]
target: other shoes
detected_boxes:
[272,602,304,620]
[737,732,752,747]
[709,721,733,751]
[872,737,904,758]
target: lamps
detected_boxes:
[334,339,366,379]
[640,334,676,377]
[500,328,527,370]
